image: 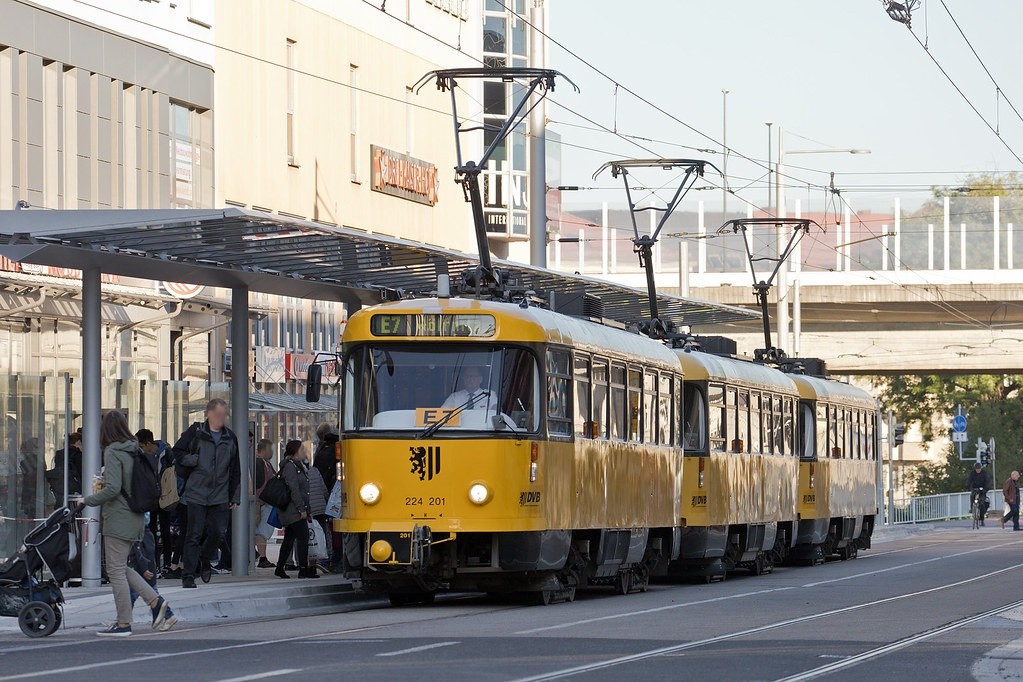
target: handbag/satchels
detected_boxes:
[266,506,283,529]
[258,462,298,510]
[175,422,203,497]
[294,519,329,562]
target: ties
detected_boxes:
[468,394,473,409]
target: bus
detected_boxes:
[334,298,880,605]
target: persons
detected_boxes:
[126,512,178,631]
[440,367,503,412]
[77,411,168,637]
[19,398,362,587]
[998,471,1023,531]
[965,463,989,520]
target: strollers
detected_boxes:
[0,502,86,637]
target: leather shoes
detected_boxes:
[182,579,197,588]
[201,564,212,583]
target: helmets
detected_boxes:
[973,463,982,468]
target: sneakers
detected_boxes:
[151,596,168,629]
[158,617,178,631]
[95,623,132,636]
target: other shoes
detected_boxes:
[969,509,972,513]
[1013,528,1023,531]
[157,561,183,579]
[284,565,300,571]
[298,566,320,579]
[999,519,1004,529]
[274,568,290,578]
[980,521,985,526]
[211,561,232,574]
[258,556,275,567]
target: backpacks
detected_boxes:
[47,450,81,500]
[159,465,179,509]
[120,448,161,513]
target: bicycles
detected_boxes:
[972,493,983,531]
